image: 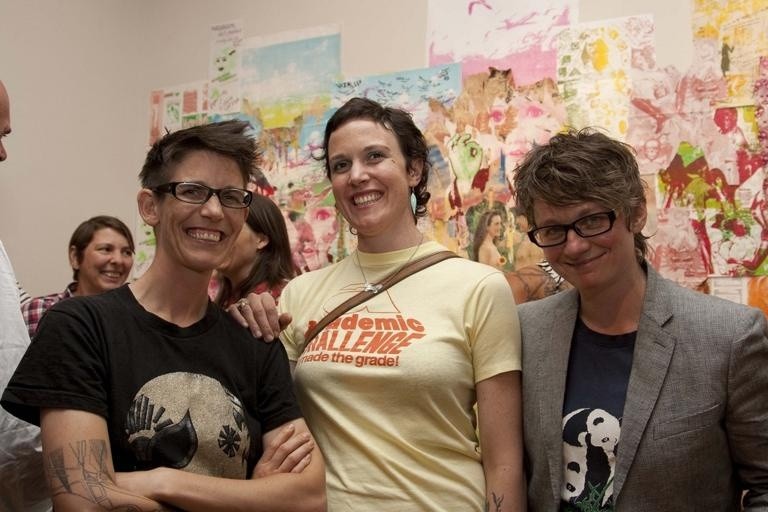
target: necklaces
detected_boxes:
[356,231,423,293]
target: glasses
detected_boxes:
[148,182,252,209]
[527,202,624,248]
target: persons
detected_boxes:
[224,98,527,512]
[0,82,54,512]
[213,192,295,312]
[513,127,768,512]
[474,211,502,269]
[0,119,327,512]
[20,215,135,341]
[285,181,339,272]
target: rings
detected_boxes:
[238,301,249,311]
